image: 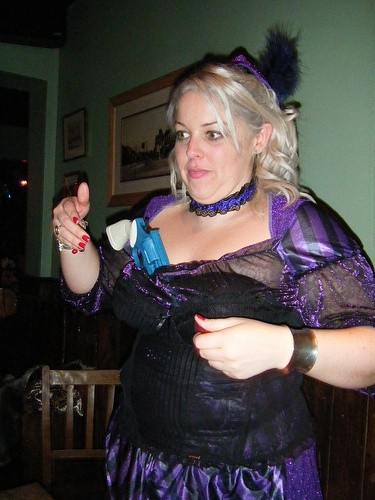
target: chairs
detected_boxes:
[41,365,120,486]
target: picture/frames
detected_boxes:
[63,170,80,188]
[106,60,199,209]
[61,106,88,162]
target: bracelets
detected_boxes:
[55,218,88,250]
[277,322,318,375]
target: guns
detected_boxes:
[107,216,170,275]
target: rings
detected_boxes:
[54,226,59,234]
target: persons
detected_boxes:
[51,60,375,500]
[0,270,19,318]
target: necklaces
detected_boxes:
[189,175,256,217]
